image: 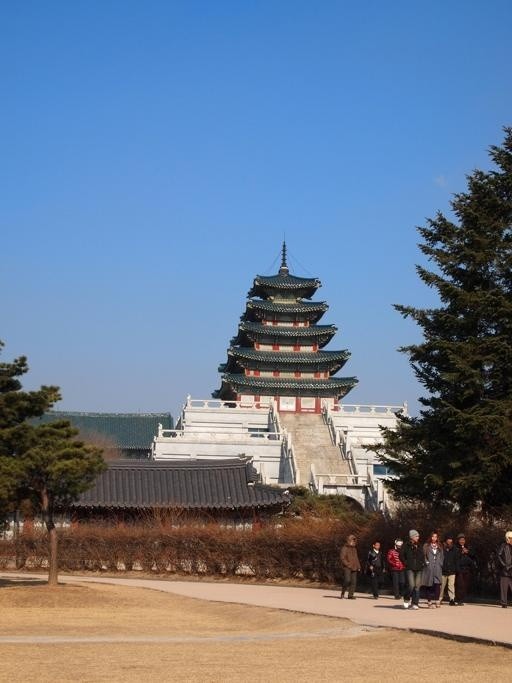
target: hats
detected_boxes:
[409,529,419,538]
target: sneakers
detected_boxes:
[502,604,507,608]
[339,594,464,609]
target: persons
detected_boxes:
[454,532,480,604]
[384,538,407,601]
[437,534,459,605]
[336,532,361,598]
[491,529,512,608]
[363,540,386,598]
[397,528,426,609]
[421,529,445,604]
